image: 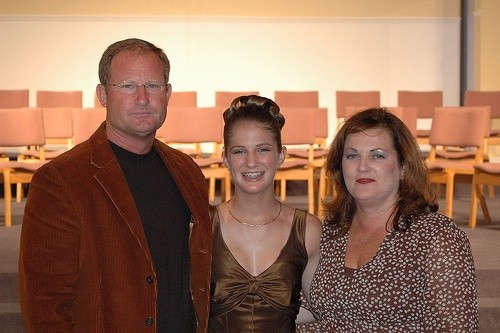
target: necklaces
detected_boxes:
[228,199,282,227]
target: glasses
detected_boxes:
[104,80,166,95]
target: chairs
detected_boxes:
[0,90,500,230]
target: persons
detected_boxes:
[207,95,324,333]
[294,108,479,333]
[19,39,213,333]
